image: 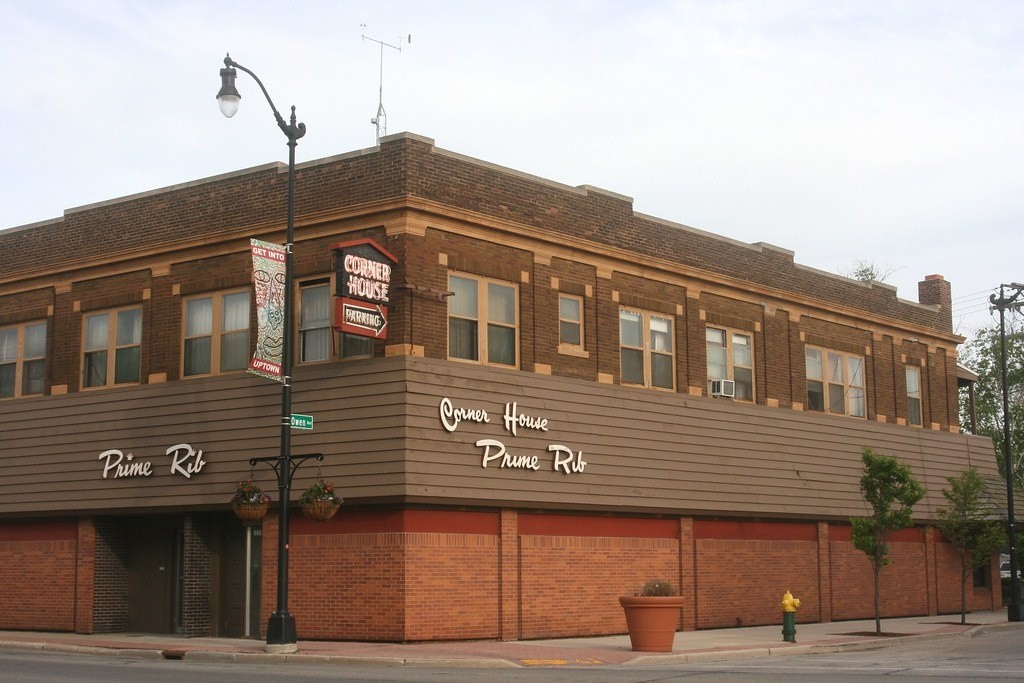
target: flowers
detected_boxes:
[300,480,344,505]
[229,479,273,509]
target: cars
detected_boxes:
[1000,562,1022,579]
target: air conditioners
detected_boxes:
[709,379,734,398]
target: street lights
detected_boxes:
[218,53,325,657]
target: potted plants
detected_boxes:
[619,580,685,652]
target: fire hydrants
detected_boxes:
[781,589,805,644]
